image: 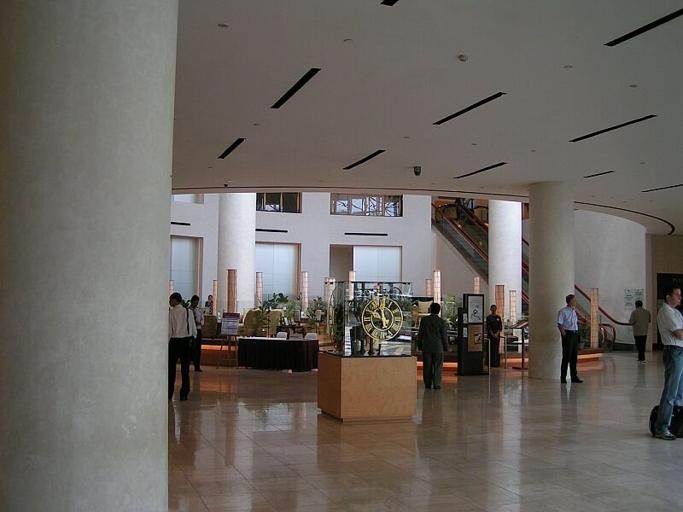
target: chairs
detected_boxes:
[276,332,317,340]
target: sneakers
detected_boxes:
[424,385,442,390]
[654,428,678,441]
[194,368,203,373]
[168,394,189,402]
[560,376,584,384]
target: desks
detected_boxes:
[238,336,319,372]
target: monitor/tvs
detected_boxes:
[198,307,210,315]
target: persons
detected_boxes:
[187,294,204,373]
[454,197,464,222]
[466,198,475,221]
[627,299,652,361]
[416,301,449,390]
[167,293,198,401]
[203,295,212,313]
[653,282,682,441]
[483,304,503,368]
[556,294,584,383]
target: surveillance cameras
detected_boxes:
[414,166,422,175]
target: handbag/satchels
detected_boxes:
[648,404,683,438]
